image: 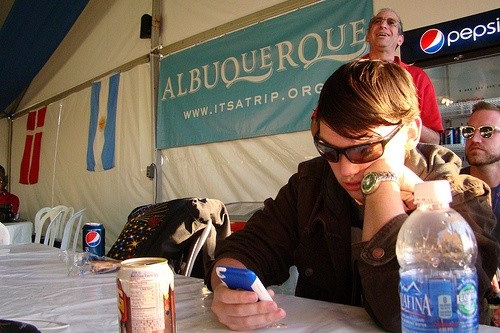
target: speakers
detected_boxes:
[139,14,152,39]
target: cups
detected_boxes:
[63,244,90,277]
[14,210,20,220]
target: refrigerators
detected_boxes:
[397,7,500,171]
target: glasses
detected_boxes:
[461,126,500,139]
[370,15,401,27]
[312,124,405,164]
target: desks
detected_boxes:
[3,220,33,245]
[0,243,500,333]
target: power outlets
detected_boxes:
[147,166,154,178]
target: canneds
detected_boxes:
[82,222,105,264]
[116,257,178,333]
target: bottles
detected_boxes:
[396,180,480,333]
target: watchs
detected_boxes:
[360,171,401,201]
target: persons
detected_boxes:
[203,58,500,333]
[0,164,20,223]
[459,101,500,293]
[310,7,444,145]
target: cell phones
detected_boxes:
[216,266,273,302]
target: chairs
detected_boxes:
[34,207,54,248]
[35,205,67,247]
[60,209,87,251]
[53,207,74,249]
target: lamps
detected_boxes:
[140,13,151,39]
